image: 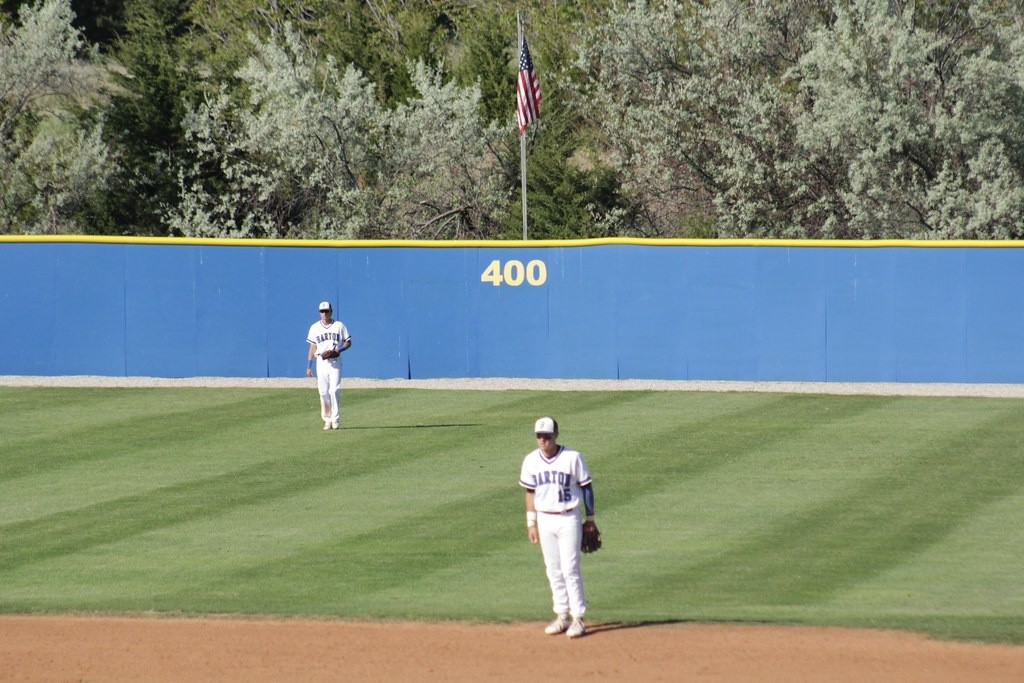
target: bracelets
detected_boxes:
[526,511,537,527]
[306,360,312,369]
[338,346,345,352]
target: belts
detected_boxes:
[542,508,573,514]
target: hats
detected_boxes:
[319,301,331,311]
[535,418,558,434]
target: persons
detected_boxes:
[305,301,352,429]
[518,416,602,638]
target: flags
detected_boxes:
[516,25,543,135]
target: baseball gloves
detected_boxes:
[322,350,339,360]
[580,521,602,553]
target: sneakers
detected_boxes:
[546,617,570,634]
[567,619,584,637]
[323,421,339,429]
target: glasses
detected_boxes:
[320,310,331,313]
